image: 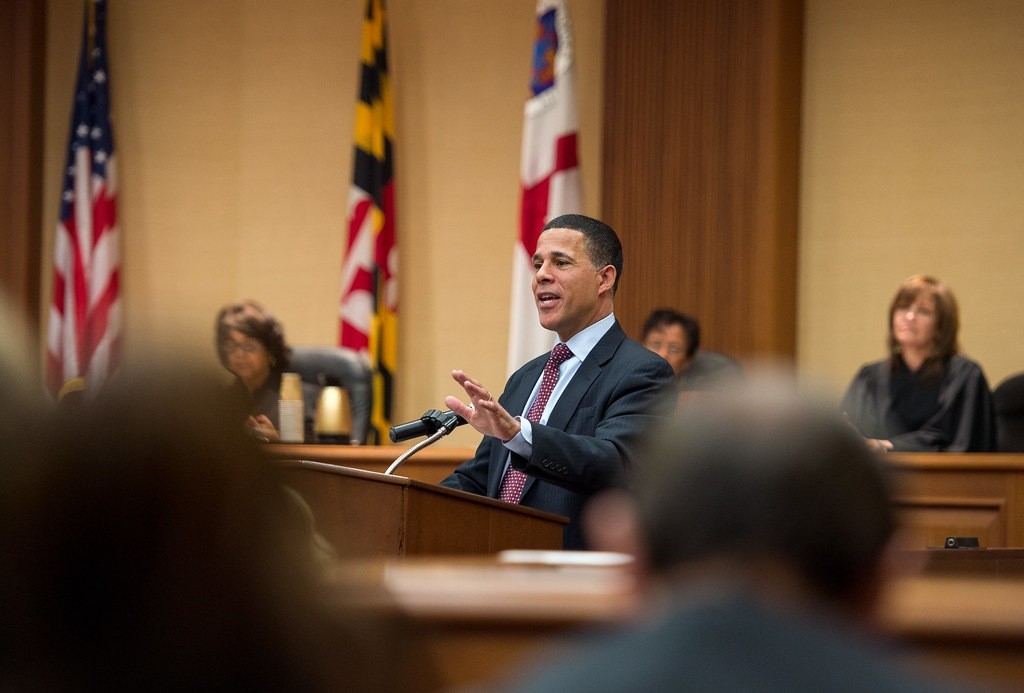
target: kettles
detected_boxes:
[312,373,353,445]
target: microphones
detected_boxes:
[389,401,483,443]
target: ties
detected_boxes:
[496,342,574,506]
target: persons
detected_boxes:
[442,214,678,553]
[212,300,327,445]
[489,367,952,693]
[639,307,701,379]
[830,275,1002,460]
[6,339,450,693]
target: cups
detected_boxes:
[275,373,307,445]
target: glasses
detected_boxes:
[221,337,261,354]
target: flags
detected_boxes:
[333,2,400,446]
[504,0,592,397]
[47,0,129,411]
